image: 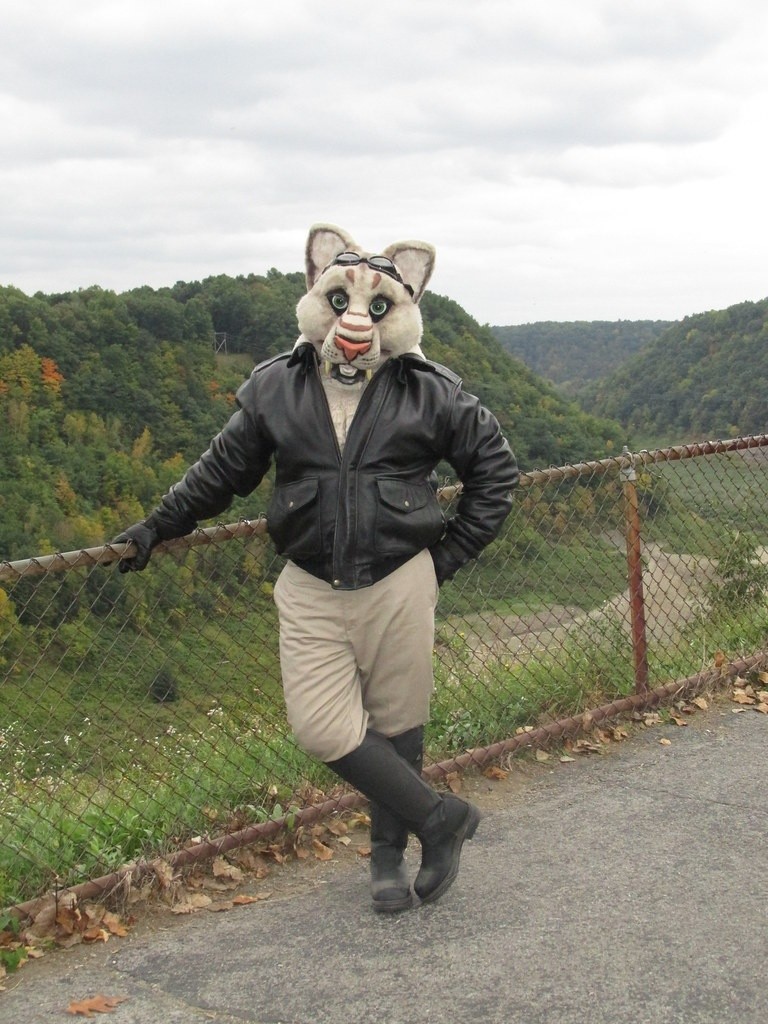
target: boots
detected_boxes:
[370,723,423,913]
[322,728,481,903]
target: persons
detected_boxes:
[101,223,519,913]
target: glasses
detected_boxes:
[310,250,415,294]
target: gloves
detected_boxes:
[104,518,159,574]
[430,541,458,586]
